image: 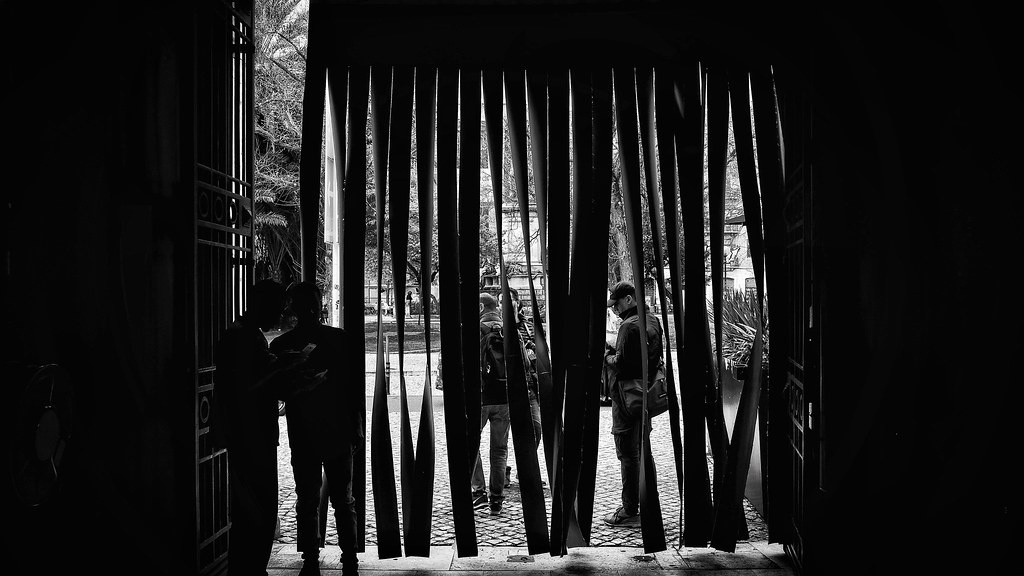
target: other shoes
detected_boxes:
[505,466,513,488]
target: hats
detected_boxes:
[608,282,635,307]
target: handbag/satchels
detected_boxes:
[617,370,669,422]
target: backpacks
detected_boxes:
[478,323,527,401]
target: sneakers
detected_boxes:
[488,501,504,516]
[603,507,642,528]
[472,491,488,510]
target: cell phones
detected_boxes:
[312,368,328,379]
[302,343,316,354]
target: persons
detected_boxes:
[269,282,364,576]
[207,281,288,576]
[471,293,514,514]
[604,281,664,526]
[497,288,546,487]
